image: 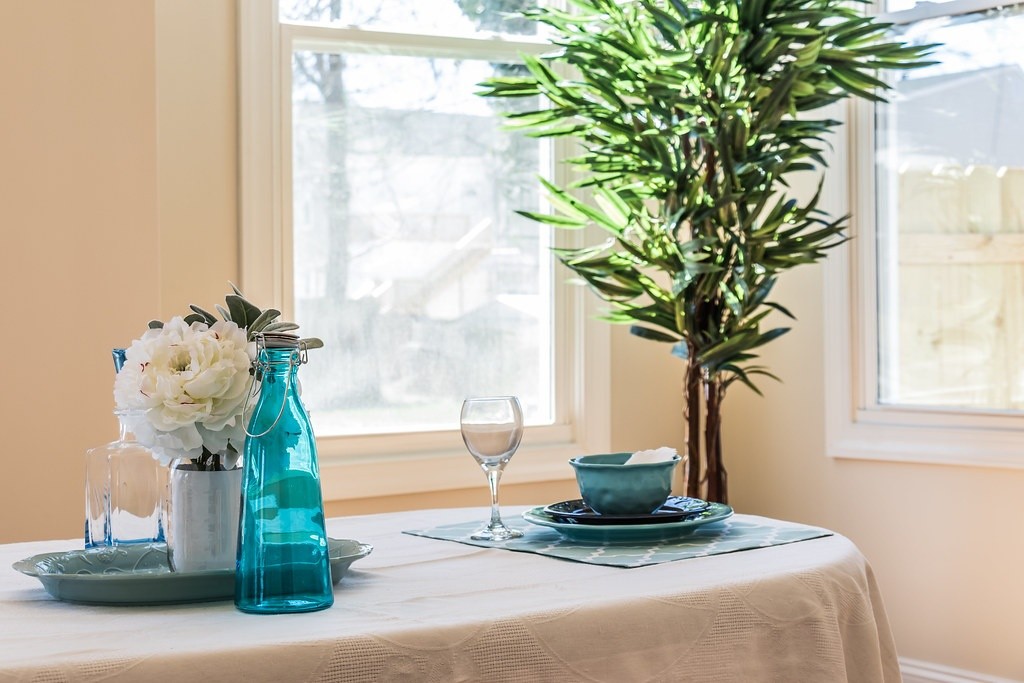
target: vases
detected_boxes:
[168,462,251,574]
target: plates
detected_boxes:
[543,495,709,524]
[521,501,735,543]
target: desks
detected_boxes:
[0,500,898,683]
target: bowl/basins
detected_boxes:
[568,452,681,514]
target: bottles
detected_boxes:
[234,332,333,614]
[86,349,168,548]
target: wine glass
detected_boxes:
[459,395,525,541]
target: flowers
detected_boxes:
[104,290,321,478]
